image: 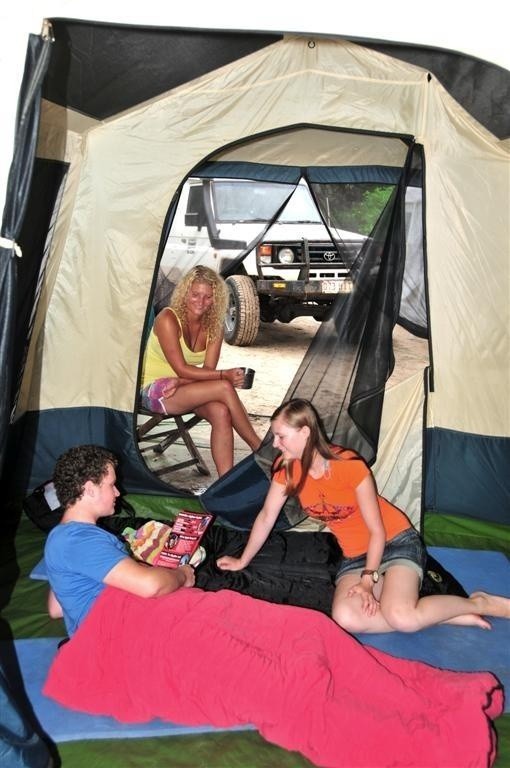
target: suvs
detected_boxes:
[154,169,390,348]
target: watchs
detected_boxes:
[359,569,380,585]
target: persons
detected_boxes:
[141,264,263,476]
[42,447,194,640]
[216,397,509,636]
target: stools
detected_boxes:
[134,406,212,477]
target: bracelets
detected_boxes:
[219,370,224,380]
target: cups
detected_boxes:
[237,367,255,390]
